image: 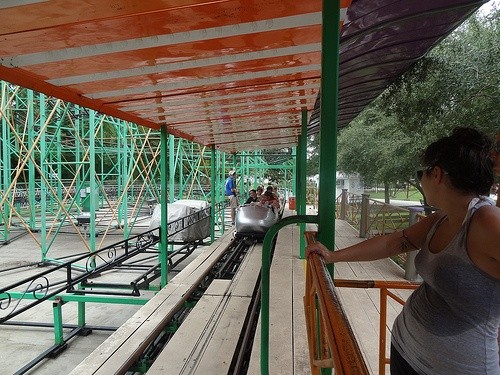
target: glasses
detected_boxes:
[416,166,434,180]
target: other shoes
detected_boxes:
[231,222,235,225]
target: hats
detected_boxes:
[228,171,235,175]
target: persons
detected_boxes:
[245,178,281,215]
[225,170,239,225]
[488,130,500,207]
[303,126,500,375]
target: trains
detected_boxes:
[232,198,287,243]
